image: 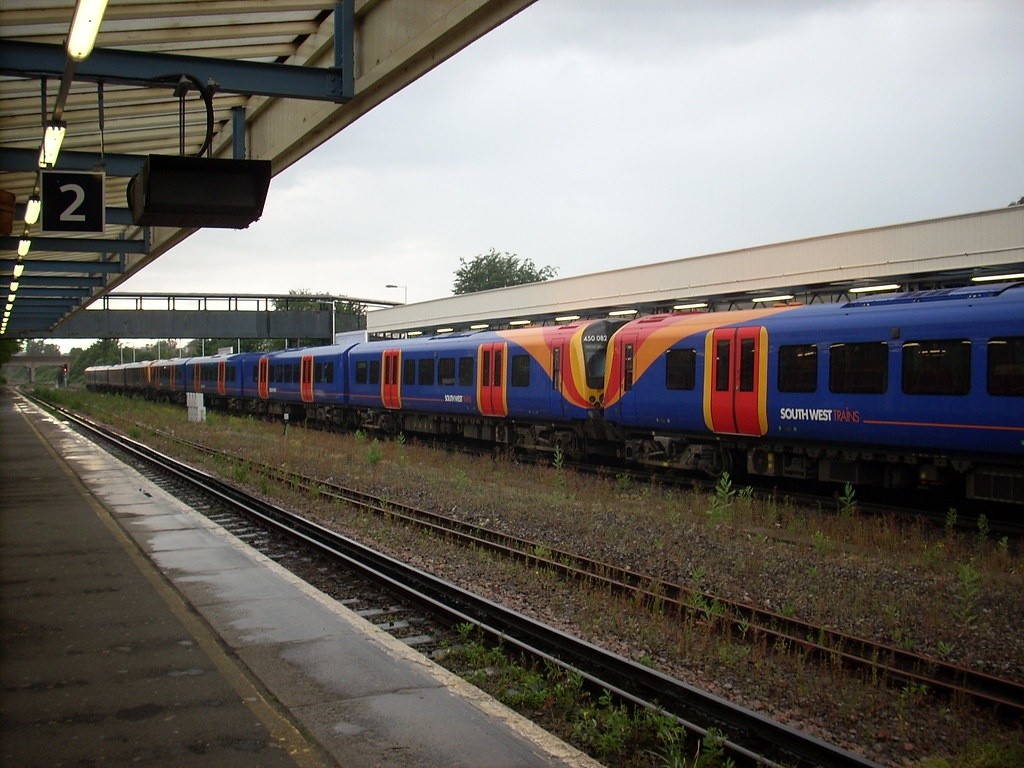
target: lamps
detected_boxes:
[36,120,67,170]
[65,0,108,63]
[407,272,1024,336]
[17,236,31,256]
[0,262,24,335]
[23,194,42,225]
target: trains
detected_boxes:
[83,275,1024,535]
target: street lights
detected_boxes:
[386,285,407,303]
